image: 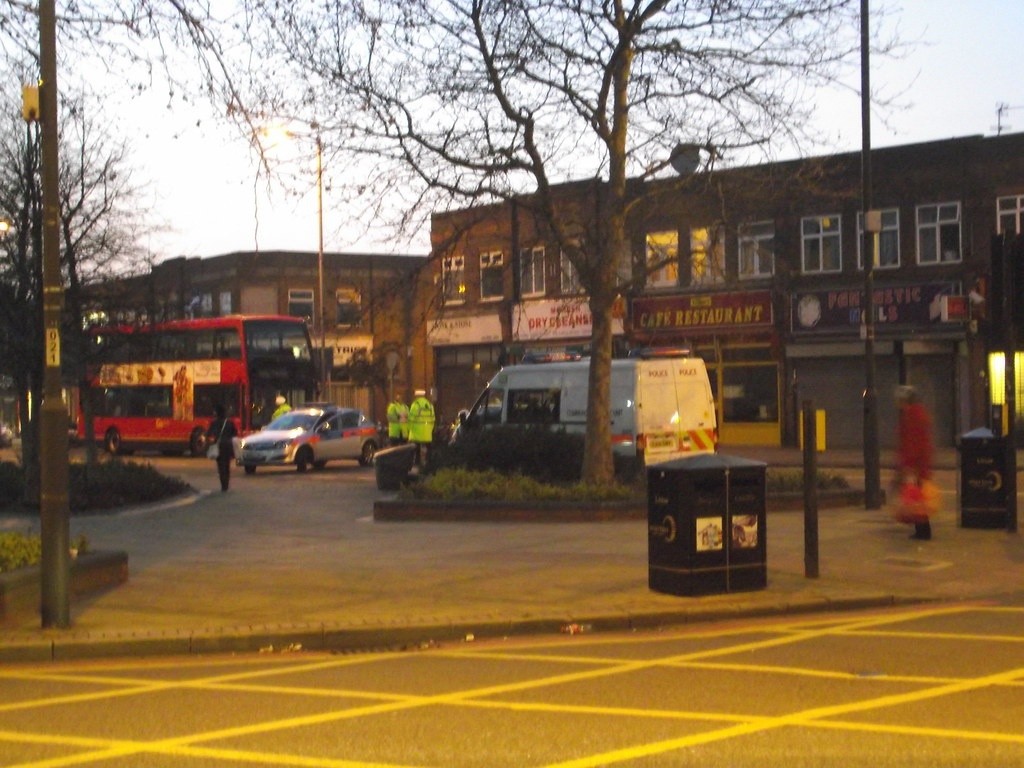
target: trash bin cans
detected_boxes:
[650,455,769,596]
[960,428,1008,528]
[371,444,417,489]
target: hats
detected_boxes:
[415,389,426,396]
[275,396,286,405]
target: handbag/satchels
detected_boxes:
[207,443,219,459]
[891,475,941,525]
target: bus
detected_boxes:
[72,313,319,456]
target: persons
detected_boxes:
[891,385,935,541]
[271,396,291,423]
[388,393,411,447]
[407,389,436,465]
[206,404,238,492]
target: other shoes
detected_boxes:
[909,523,931,540]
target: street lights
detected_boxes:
[265,123,326,398]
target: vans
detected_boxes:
[444,350,719,465]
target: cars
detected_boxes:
[237,403,379,475]
[0,423,22,467]
